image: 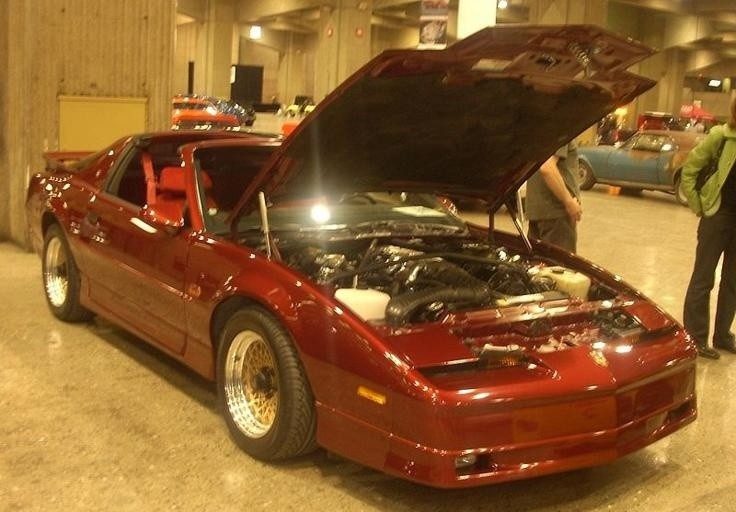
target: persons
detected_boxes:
[596,113,727,147]
[524,137,582,254]
[680,88,736,359]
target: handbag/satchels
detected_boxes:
[692,159,720,191]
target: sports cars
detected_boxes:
[25,24,700,490]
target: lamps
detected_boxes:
[250,25,261,40]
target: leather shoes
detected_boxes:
[692,342,722,362]
[712,334,736,354]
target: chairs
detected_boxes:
[154,168,217,223]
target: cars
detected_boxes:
[173,96,248,130]
[579,111,717,209]
